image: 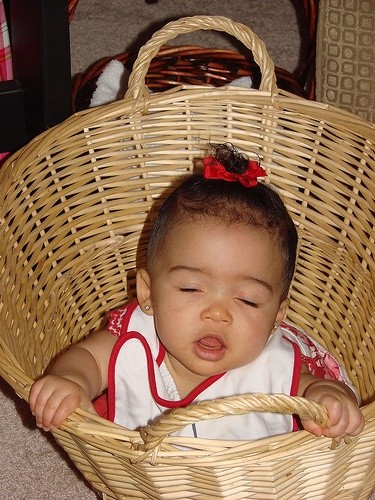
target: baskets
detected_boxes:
[67,0,321,115]
[0,15,374,500]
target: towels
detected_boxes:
[88,59,251,197]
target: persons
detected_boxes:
[29,142,365,441]
